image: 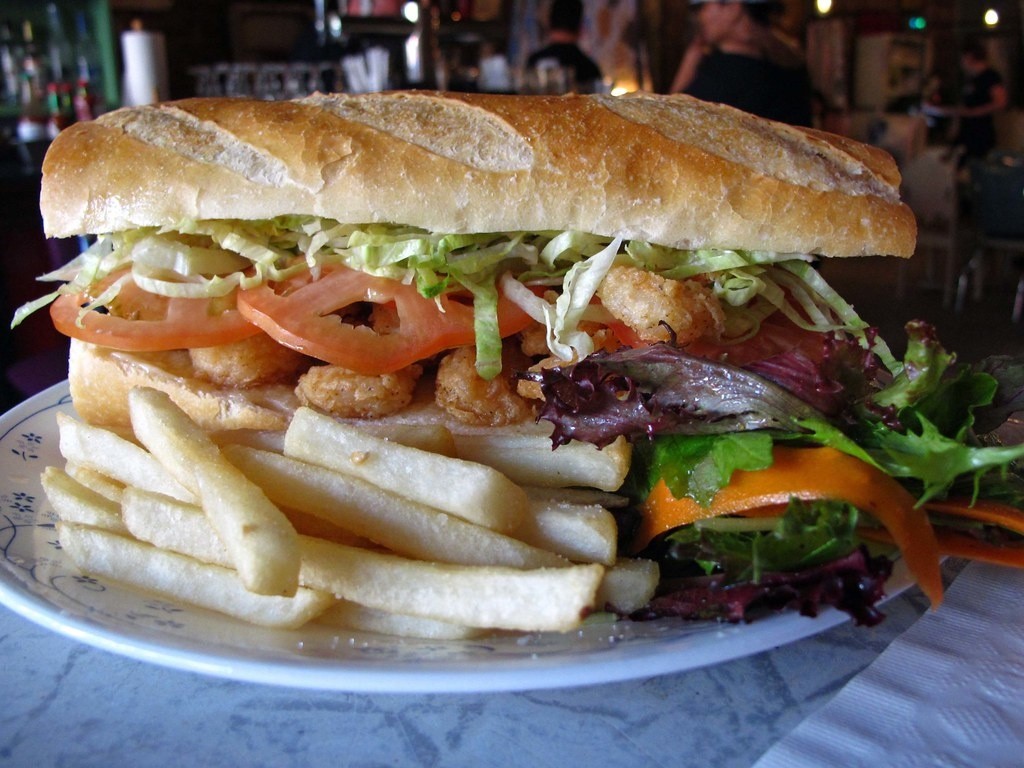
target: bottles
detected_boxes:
[2,39,103,183]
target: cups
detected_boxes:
[182,59,347,105]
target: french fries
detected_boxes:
[38,385,660,633]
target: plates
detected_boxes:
[0,374,958,698]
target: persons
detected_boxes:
[815,60,949,150]
[672,1,812,127]
[949,39,1010,156]
[523,0,602,97]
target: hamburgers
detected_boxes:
[12,91,1024,629]
